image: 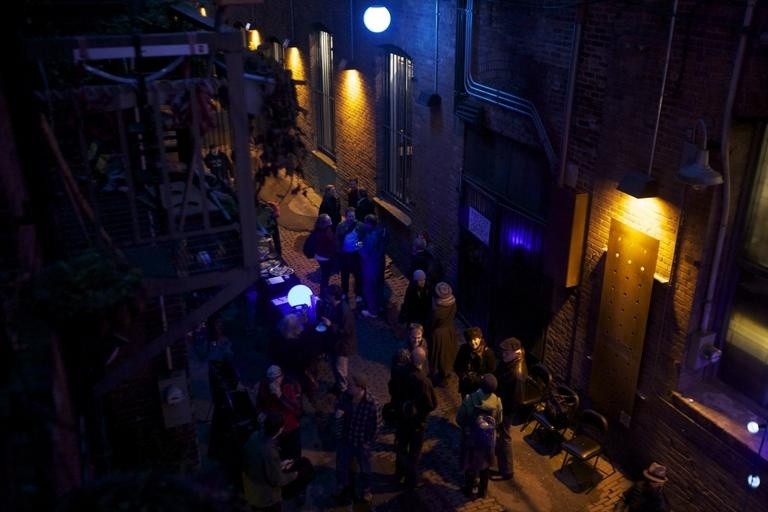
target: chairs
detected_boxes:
[506,361,619,486]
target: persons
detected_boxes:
[194,61,302,182]
[616,462,671,512]
[242,170,528,511]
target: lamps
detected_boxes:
[413,2,441,110]
[190,0,367,86]
[741,418,768,492]
[672,115,729,194]
[360,1,392,34]
[615,0,698,201]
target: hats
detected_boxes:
[500,338,521,350]
[414,270,425,280]
[436,282,452,298]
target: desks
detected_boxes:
[244,228,325,344]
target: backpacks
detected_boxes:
[474,413,497,446]
